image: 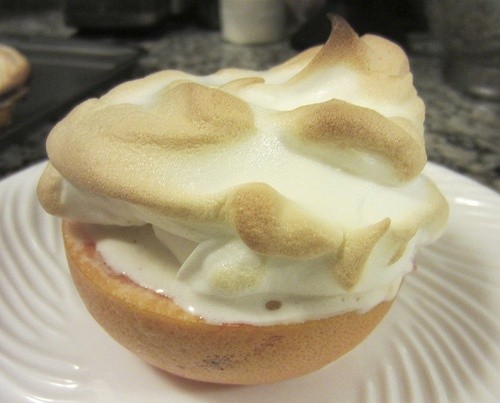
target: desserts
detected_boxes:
[36,13,450,387]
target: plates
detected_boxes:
[0,155,499,401]
